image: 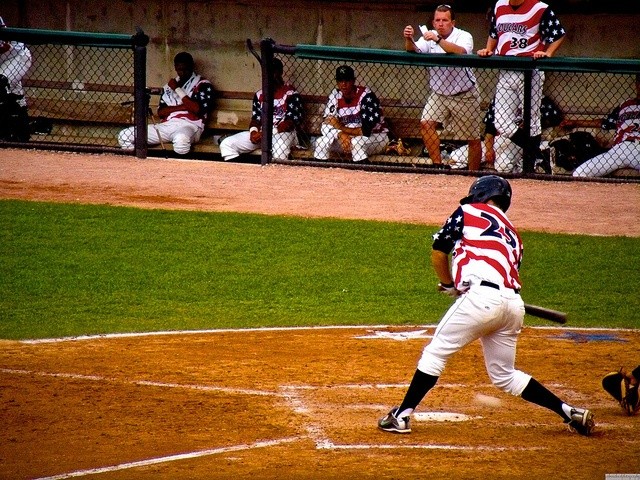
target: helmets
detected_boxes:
[460,175,513,213]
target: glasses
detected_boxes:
[437,4,453,9]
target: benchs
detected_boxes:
[18,77,640,183]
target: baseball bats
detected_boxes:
[437,282,567,324]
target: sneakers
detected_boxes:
[563,407,597,436]
[604,372,640,415]
[379,406,412,433]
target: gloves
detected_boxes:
[437,282,458,299]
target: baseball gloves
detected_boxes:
[385,138,411,156]
[600,370,637,417]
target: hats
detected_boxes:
[335,65,358,81]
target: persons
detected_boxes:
[0,16,32,143]
[117,52,215,158]
[477,0,567,173]
[403,5,481,172]
[571,74,640,180]
[219,58,303,162]
[602,363,640,417]
[314,65,393,164]
[445,83,564,172]
[379,176,597,434]
